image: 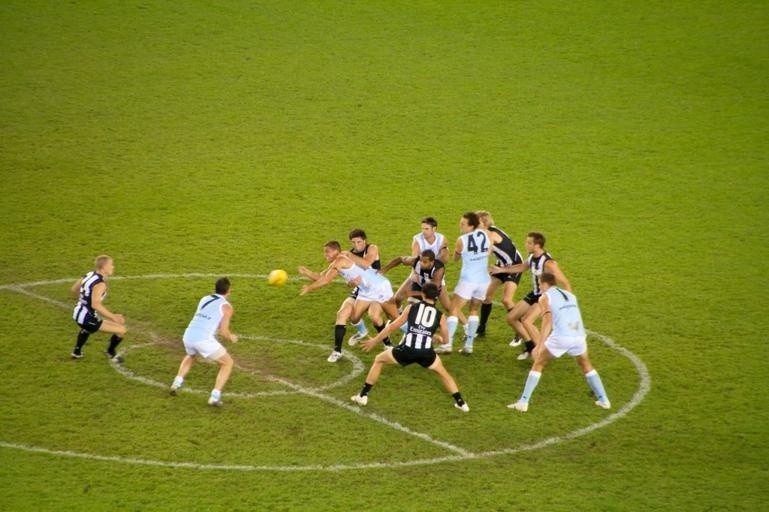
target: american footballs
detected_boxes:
[268,270,287,285]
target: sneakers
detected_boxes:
[327,332,473,363]
[350,394,368,406]
[170,384,178,396]
[509,336,523,347]
[104,347,125,363]
[506,400,529,412]
[71,350,84,358]
[454,400,470,412]
[208,395,224,407]
[475,326,485,338]
[516,344,535,360]
[594,399,611,410]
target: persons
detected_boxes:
[169,276,238,407]
[70,255,126,364]
[298,211,611,413]
